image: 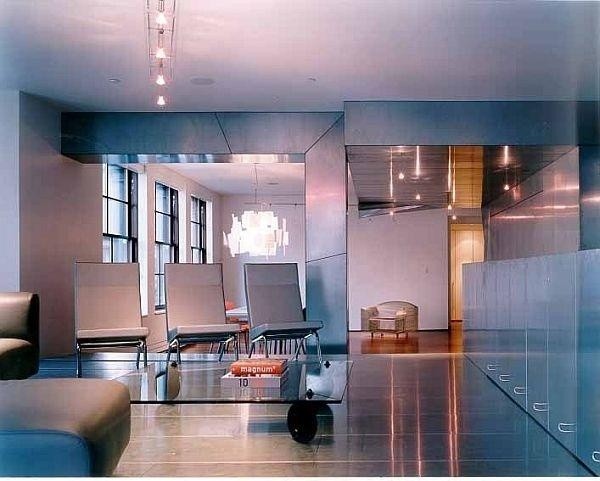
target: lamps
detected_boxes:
[222,165,291,259]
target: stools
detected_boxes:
[0,377,131,477]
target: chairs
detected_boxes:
[0,291,40,380]
[361,301,418,330]
[164,263,242,363]
[244,262,324,361]
[72,260,150,379]
[209,303,247,354]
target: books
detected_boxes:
[238,377,290,388]
[230,356,289,375]
[222,364,290,380]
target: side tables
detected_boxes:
[225,307,249,353]
